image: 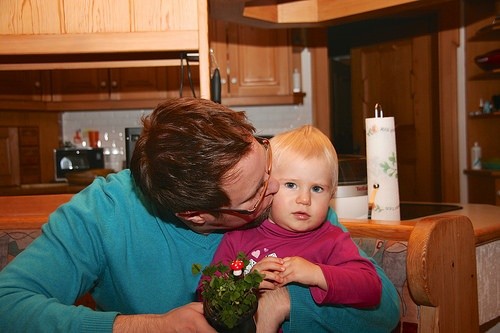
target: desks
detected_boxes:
[370,201,500,324]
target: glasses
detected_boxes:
[207,135,274,217]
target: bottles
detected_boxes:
[293,68,299,93]
[470,142,483,171]
[73,130,81,144]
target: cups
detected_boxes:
[87,130,99,149]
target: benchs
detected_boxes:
[0,214,480,333]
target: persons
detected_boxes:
[196,126,383,310]
[0,97,402,333]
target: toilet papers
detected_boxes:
[365,117,400,221]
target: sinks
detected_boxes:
[368,204,463,220]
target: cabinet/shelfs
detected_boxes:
[462,0,500,207]
[0,0,307,106]
